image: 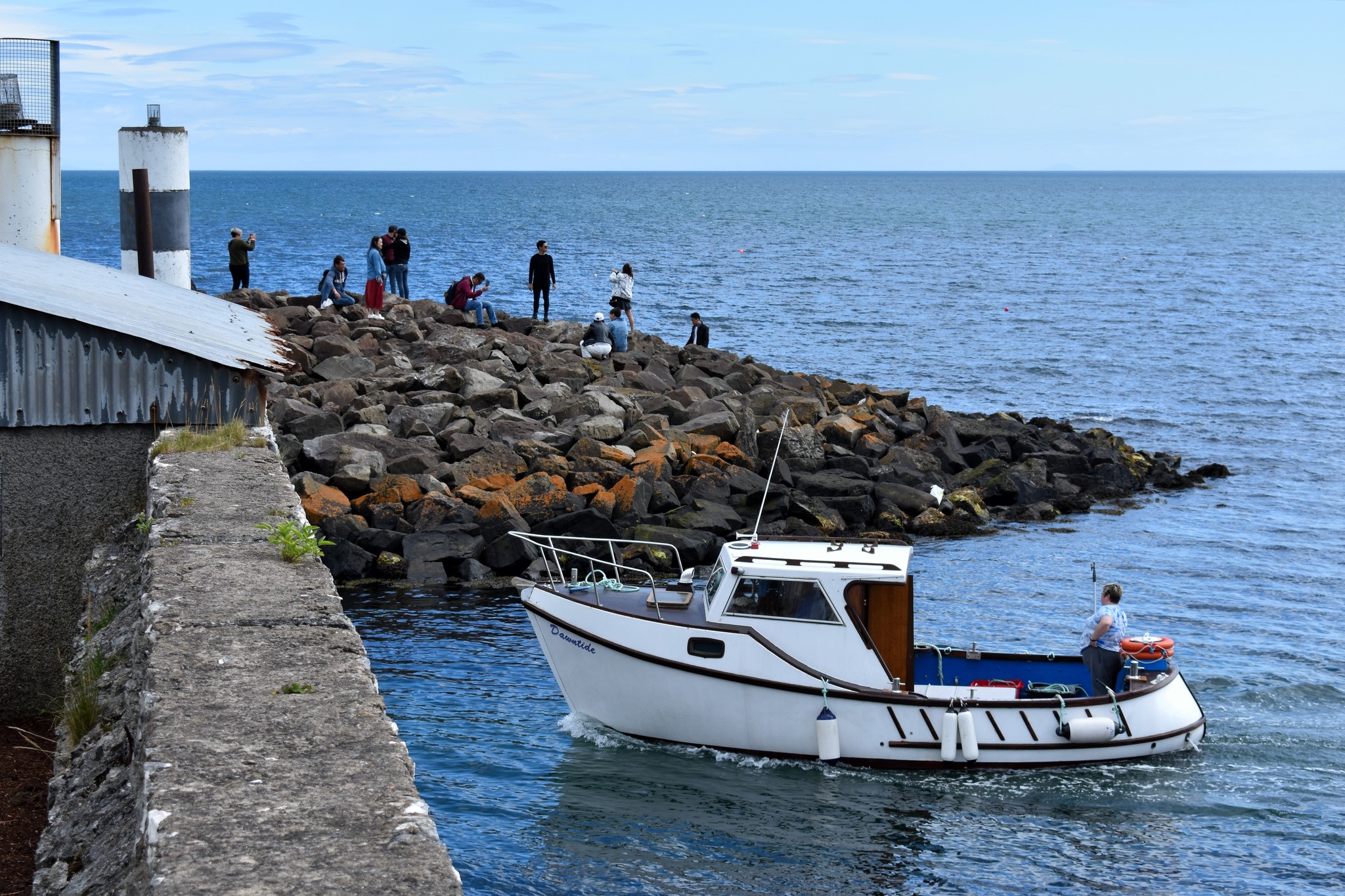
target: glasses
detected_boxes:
[477,277,482,284]
[544,246,548,249]
[338,263,346,266]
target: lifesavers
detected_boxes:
[1119,636,1175,650]
[1120,649,1176,660]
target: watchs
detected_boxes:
[1089,637,1097,641]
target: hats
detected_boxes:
[388,225,398,232]
[594,312,605,321]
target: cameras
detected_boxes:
[250,234,253,239]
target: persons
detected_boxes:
[1079,584,1128,695]
[365,225,411,320]
[529,240,556,323]
[228,228,257,292]
[451,272,505,330]
[321,256,355,311]
[685,312,709,348]
[610,264,634,331]
[580,307,629,359]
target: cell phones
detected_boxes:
[485,281,490,287]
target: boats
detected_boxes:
[503,404,1209,769]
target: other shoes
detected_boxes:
[321,301,332,309]
[491,322,503,329]
[368,314,375,319]
[374,314,385,320]
[477,325,489,331]
[531,312,538,320]
[327,298,333,305]
[602,357,607,360]
[543,315,550,322]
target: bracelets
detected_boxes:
[614,272,617,274]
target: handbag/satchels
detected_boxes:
[609,299,617,308]
[382,273,387,280]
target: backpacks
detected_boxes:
[318,269,336,292]
[445,278,472,305]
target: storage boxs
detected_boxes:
[971,680,1025,698]
[1028,682,1082,699]
